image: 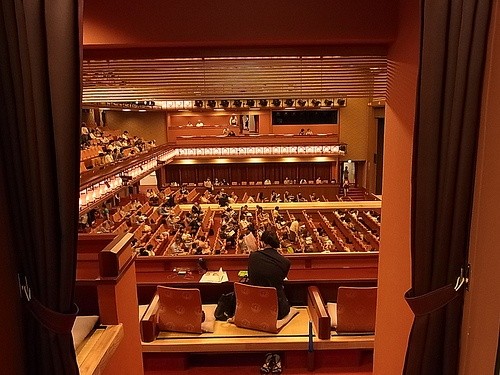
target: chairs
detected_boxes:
[327,286,377,333]
[232,282,300,333]
[153,286,203,333]
[88,180,383,254]
[80,147,103,173]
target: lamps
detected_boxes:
[194,99,346,108]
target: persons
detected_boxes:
[222,126,235,137]
[81,121,156,170]
[245,115,249,131]
[229,114,238,127]
[78,177,380,256]
[299,128,313,135]
[187,119,203,127]
[247,230,290,320]
[344,166,349,179]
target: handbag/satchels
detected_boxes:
[214,292,236,321]
